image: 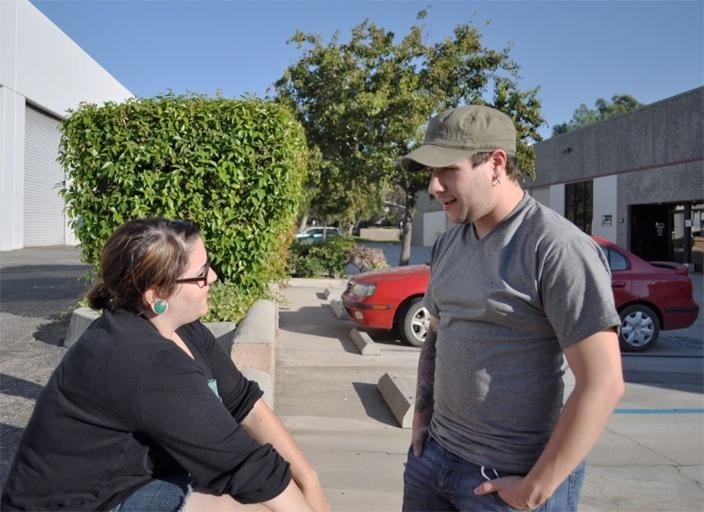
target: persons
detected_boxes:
[400,103,627,512]
[0,216,335,512]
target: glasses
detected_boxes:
[174,256,212,286]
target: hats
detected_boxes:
[398,104,517,172]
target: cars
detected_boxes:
[342,235,700,355]
[294,226,343,239]
